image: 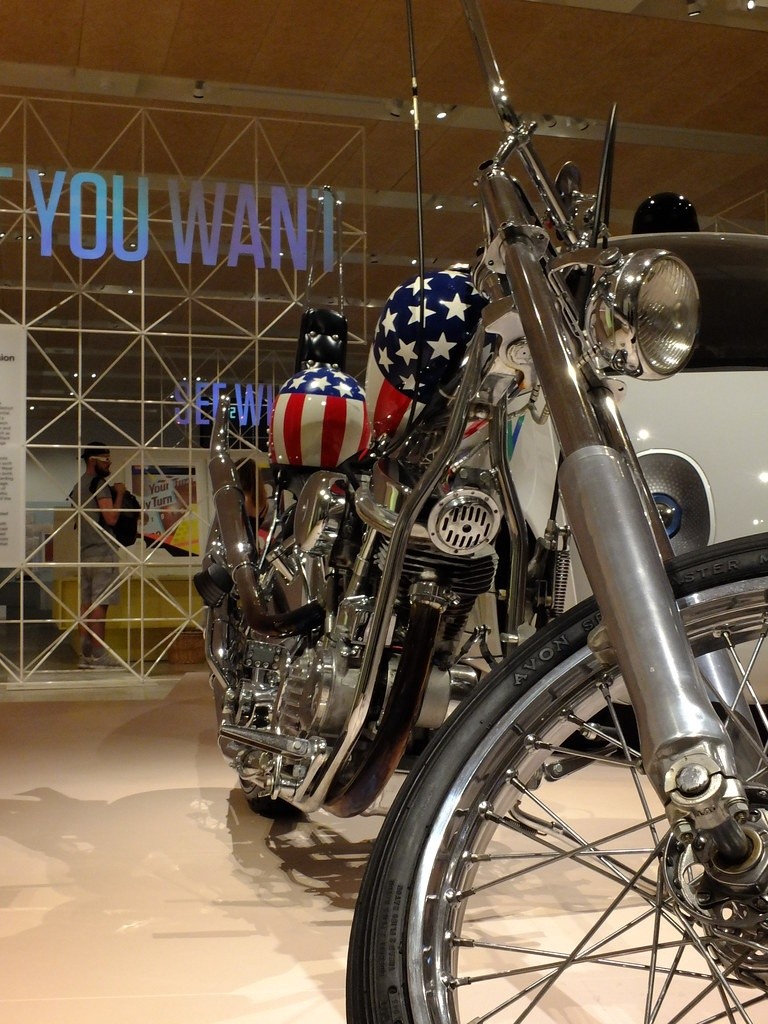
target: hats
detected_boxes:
[81,442,110,458]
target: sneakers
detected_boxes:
[78,652,95,668]
[91,654,127,671]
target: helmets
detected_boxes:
[268,367,369,474]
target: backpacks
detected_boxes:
[88,475,141,547]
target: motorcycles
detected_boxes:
[183,0,768,1024]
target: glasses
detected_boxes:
[90,455,111,463]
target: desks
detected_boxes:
[52,574,204,663]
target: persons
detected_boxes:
[70,441,126,671]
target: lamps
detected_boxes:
[346,298,355,305]
[431,194,448,211]
[686,0,701,17]
[371,254,378,263]
[572,117,590,132]
[407,257,416,263]
[726,0,755,12]
[434,105,447,119]
[388,98,403,117]
[430,258,437,264]
[264,292,272,300]
[247,291,255,300]
[363,299,371,305]
[14,231,22,240]
[126,286,134,296]
[541,115,556,128]
[129,240,137,248]
[192,81,205,98]
[27,231,34,241]
[328,297,334,304]
